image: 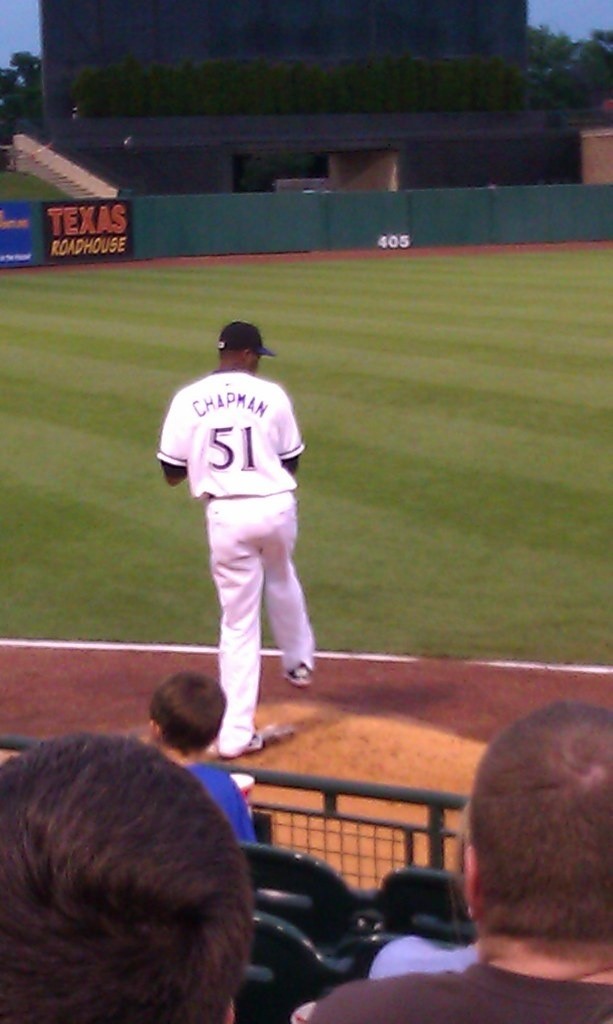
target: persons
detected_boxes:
[156,321,314,758]
[148,670,258,842]
[306,701,613,1024]
[0,733,255,1024]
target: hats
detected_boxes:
[221,321,276,356]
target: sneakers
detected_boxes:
[241,733,265,753]
[285,664,311,686]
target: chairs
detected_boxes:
[214,841,475,1024]
